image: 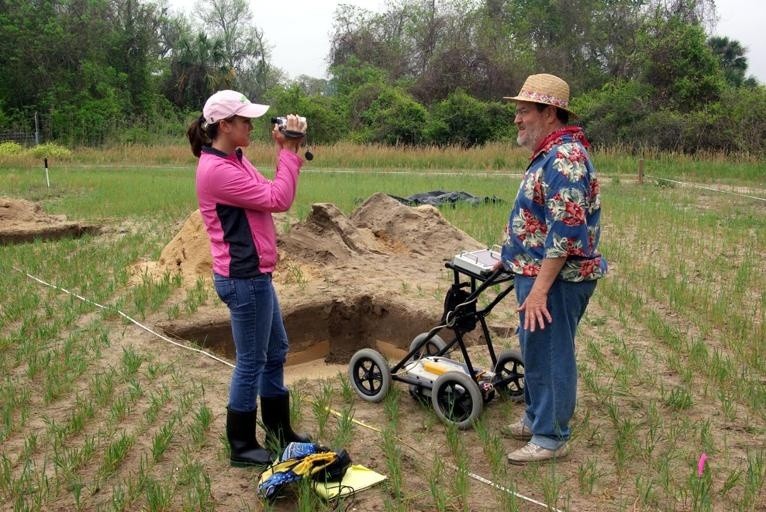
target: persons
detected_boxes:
[491,71,607,466]
[185,89,314,466]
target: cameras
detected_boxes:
[271,116,306,132]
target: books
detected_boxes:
[311,464,388,503]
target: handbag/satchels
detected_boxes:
[256,442,351,508]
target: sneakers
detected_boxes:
[507,441,572,465]
[499,419,533,439]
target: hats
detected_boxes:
[502,74,579,119]
[202,89,268,125]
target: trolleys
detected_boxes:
[346,243,527,431]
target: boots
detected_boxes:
[260,396,312,449]
[226,406,277,468]
